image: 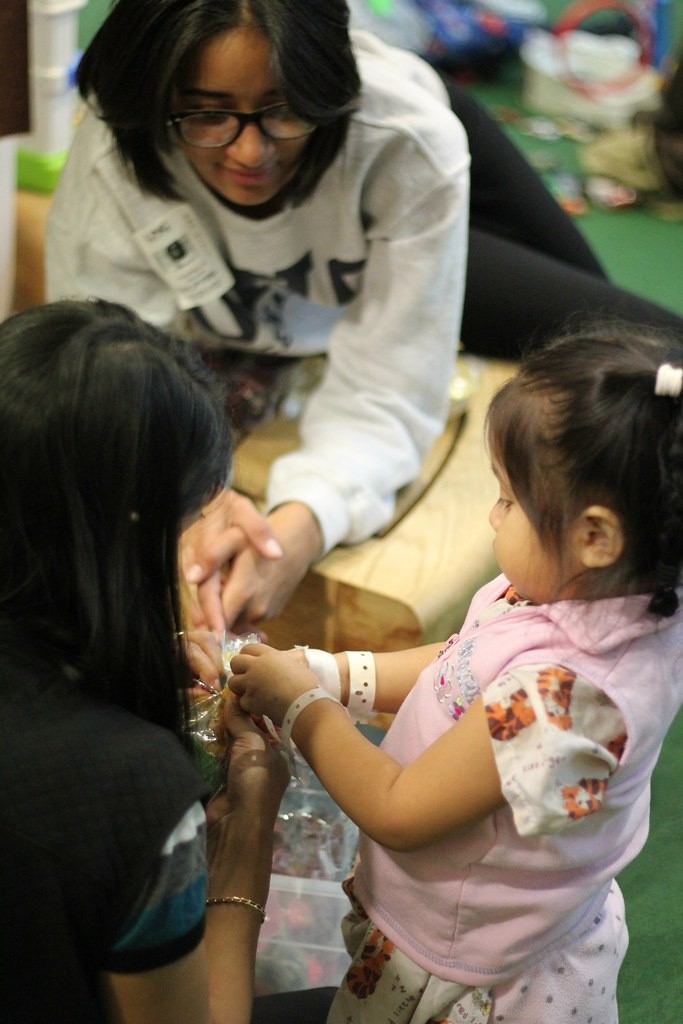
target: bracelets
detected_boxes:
[344,651,376,727]
[262,688,351,786]
[206,895,266,925]
[301,647,342,701]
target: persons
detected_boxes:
[0,295,290,1024]
[226,321,682,1024]
[41,1,682,639]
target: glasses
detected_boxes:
[163,95,318,149]
